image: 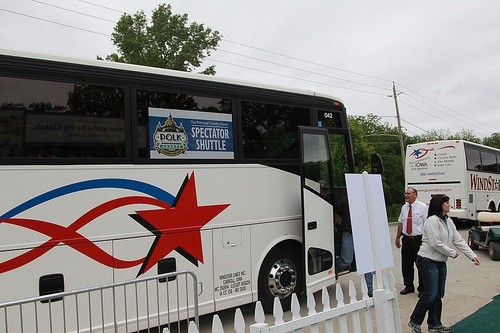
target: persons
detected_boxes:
[394,186,429,294]
[408,194,481,333]
[335,193,374,299]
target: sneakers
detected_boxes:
[428,324,451,333]
[407,320,422,333]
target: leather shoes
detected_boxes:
[400,287,415,295]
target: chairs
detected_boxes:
[477,211,500,231]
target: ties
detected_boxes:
[406,204,412,235]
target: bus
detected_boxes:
[0,51,356,331]
[405,139,500,226]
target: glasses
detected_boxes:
[442,194,446,200]
[403,193,412,195]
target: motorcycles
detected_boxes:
[468,210,500,261]
